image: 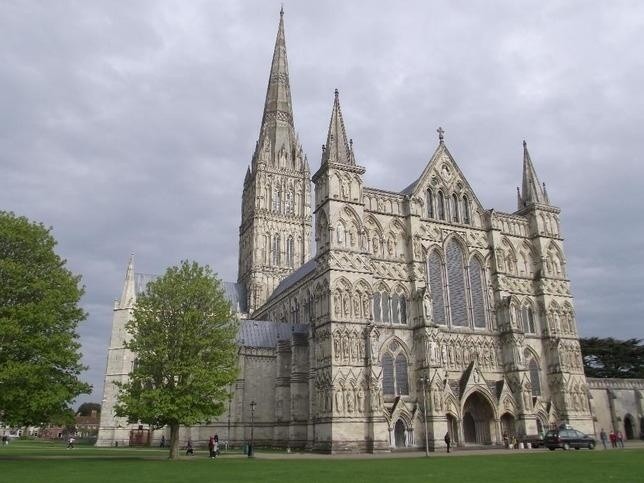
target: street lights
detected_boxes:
[419,370,429,457]
[248,400,256,456]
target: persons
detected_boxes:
[214,432,220,456]
[503,431,509,448]
[599,428,608,448]
[186,436,194,455]
[160,436,166,448]
[608,431,617,448]
[445,432,451,453]
[616,431,624,447]
[66,437,75,448]
[208,436,216,463]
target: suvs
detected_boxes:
[543,429,595,450]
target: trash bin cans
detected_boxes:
[244,445,249,454]
